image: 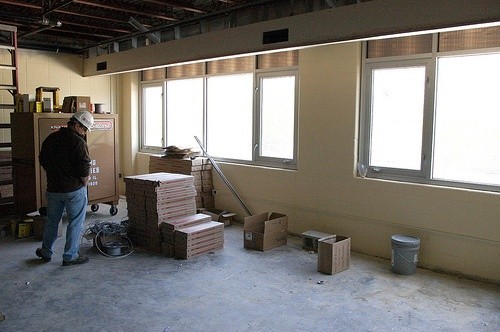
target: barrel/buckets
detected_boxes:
[391,234,421,275]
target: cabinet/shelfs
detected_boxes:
[10,114,119,216]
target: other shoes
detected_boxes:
[63,256,89,266]
[36,247,51,262]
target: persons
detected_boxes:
[36,111,94,266]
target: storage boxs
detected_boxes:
[244,210,288,253]
[123,147,237,260]
[14,94,29,112]
[16,222,34,238]
[317,235,351,274]
[63,95,91,113]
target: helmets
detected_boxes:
[73,111,94,132]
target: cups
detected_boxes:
[95,103,106,113]
[358,161,369,178]
